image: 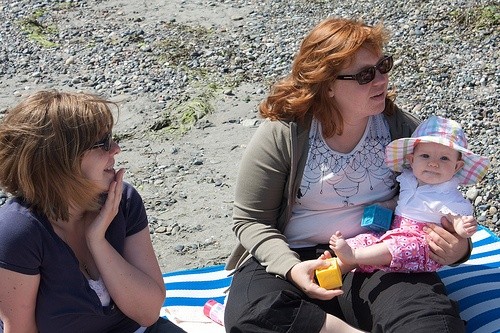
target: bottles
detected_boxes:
[203,299,224,326]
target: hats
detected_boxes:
[382,113,490,188]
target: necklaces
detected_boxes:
[52,214,95,270]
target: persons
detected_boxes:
[0,88,190,333]
[220,17,474,333]
[312,115,489,282]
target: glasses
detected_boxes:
[333,53,396,85]
[80,130,113,153]
